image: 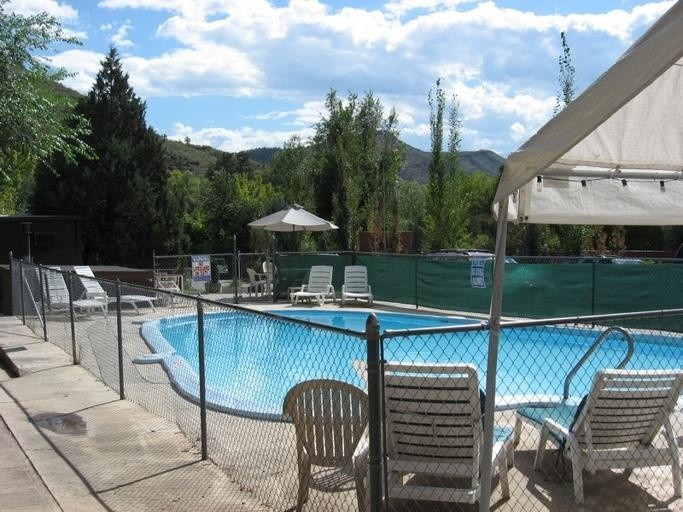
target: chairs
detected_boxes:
[33,265,182,323]
[361,496,367,509]
[280,378,369,512]
[246,261,375,307]
[510,369,682,506]
[367,362,511,512]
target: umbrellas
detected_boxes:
[246,200,340,253]
